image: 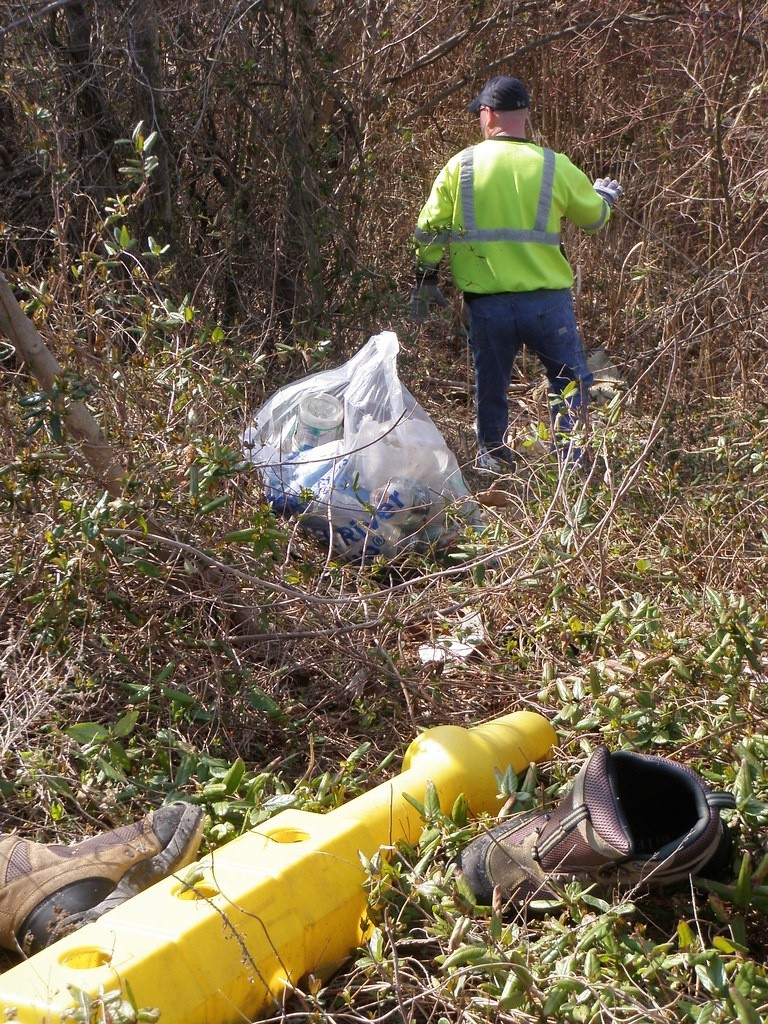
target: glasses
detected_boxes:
[475,107,485,117]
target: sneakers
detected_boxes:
[463,746,738,918]
[0,800,206,959]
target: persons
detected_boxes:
[404,75,623,507]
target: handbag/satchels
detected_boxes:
[238,329,488,567]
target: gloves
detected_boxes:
[406,263,448,330]
[592,177,623,205]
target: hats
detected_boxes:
[468,75,529,113]
